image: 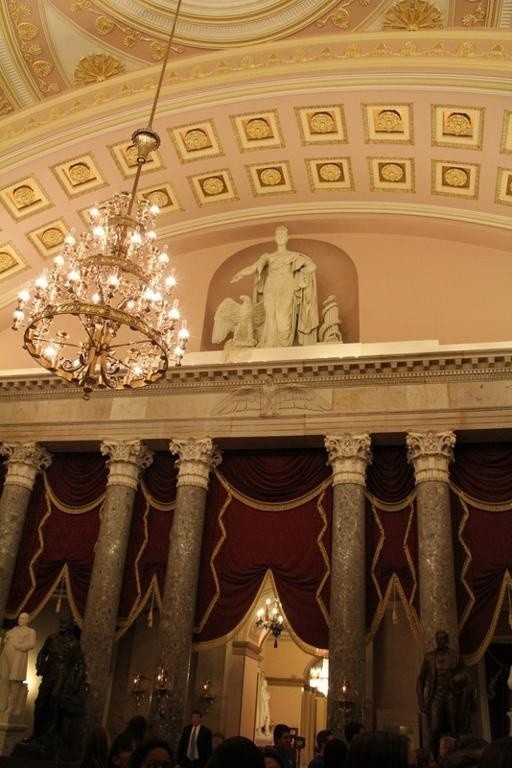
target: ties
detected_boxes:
[189,727,196,760]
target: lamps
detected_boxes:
[14,0,182,402]
[129,669,216,716]
[256,597,287,649]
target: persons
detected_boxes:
[21,611,89,747]
[308,723,510,766]
[212,724,292,766]
[230,224,319,352]
[415,630,472,754]
[1,611,37,727]
[9,709,212,766]
